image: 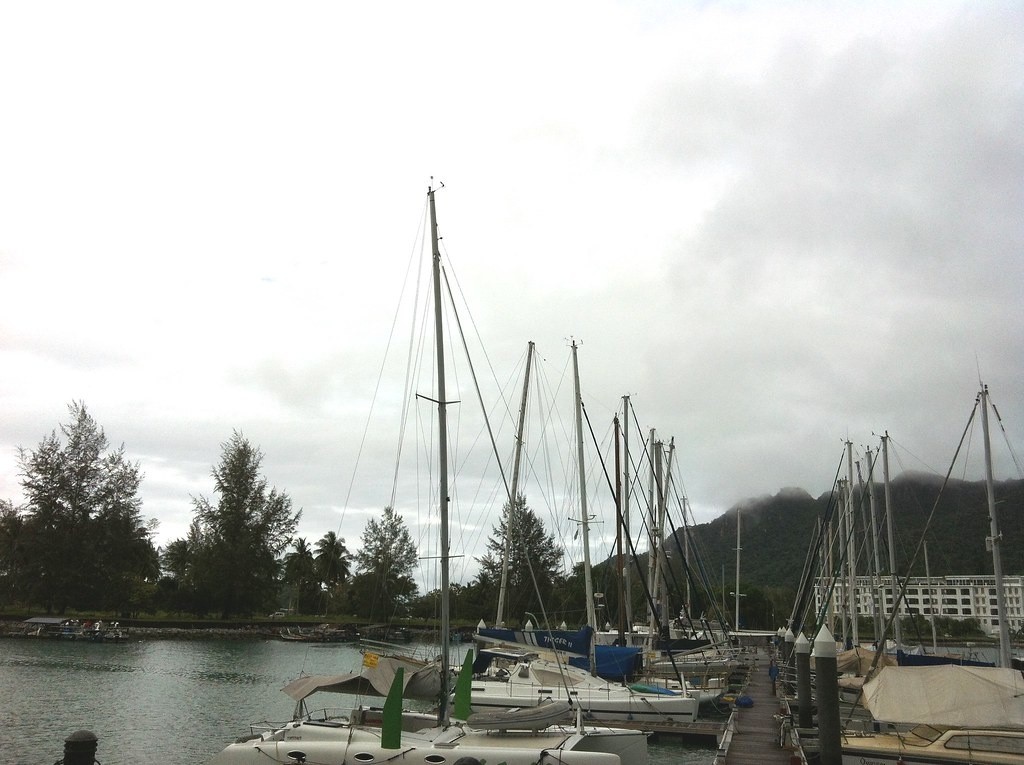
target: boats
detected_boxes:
[278,626,328,642]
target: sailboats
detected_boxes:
[206,179,735,764]
[777,381,1024,765]
